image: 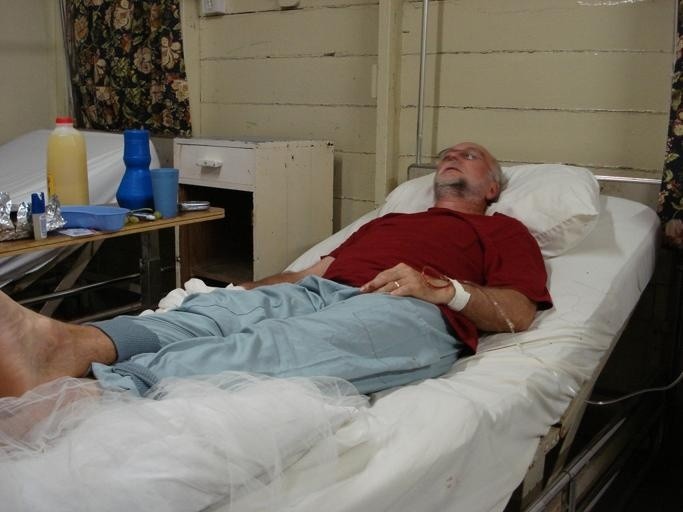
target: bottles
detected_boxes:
[31,192,48,240]
[46,117,89,208]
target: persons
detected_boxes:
[0,143,553,442]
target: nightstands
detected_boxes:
[172,138,334,287]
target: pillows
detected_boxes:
[380,164,600,257]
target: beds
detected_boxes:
[0,162,662,512]
[1,129,160,289]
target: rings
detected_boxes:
[394,281,401,289]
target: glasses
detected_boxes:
[436,146,493,172]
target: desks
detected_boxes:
[0,207,224,324]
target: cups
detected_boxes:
[150,167,179,218]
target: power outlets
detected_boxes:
[204,0,225,15]
